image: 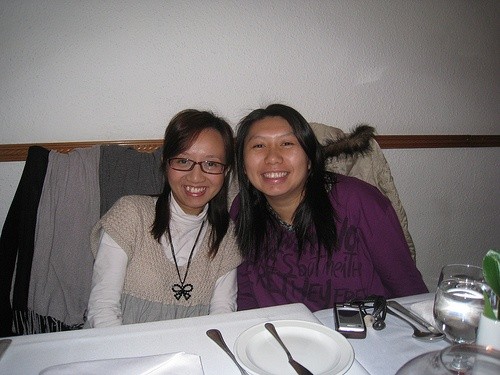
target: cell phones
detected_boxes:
[334,302,367,339]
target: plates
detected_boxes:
[233,320,355,375]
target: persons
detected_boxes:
[229,104,429,315]
[86,108,243,327]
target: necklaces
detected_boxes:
[169,220,205,300]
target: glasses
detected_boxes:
[168,157,227,175]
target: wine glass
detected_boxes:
[434,264,495,373]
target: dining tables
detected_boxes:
[0,292,452,375]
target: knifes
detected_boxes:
[265,323,314,375]
[387,299,443,338]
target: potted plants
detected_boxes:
[476,250,500,349]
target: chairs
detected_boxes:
[0,123,417,338]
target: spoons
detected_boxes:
[386,307,445,339]
